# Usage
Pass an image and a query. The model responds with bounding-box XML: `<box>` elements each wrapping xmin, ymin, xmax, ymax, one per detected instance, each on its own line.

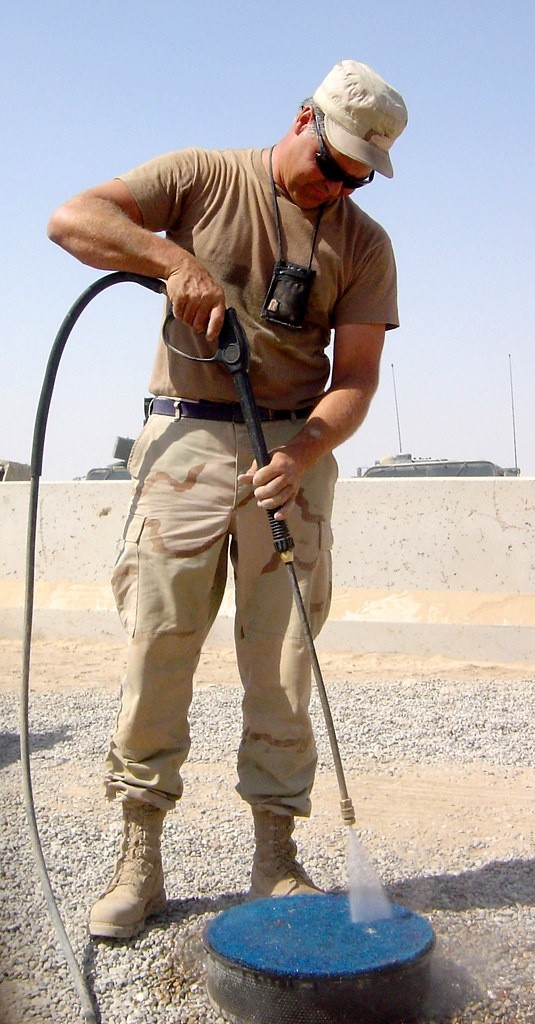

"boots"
<box><xmin>87</xmin><ymin>807</ymin><xmax>168</xmax><ymax>937</ymax></box>
<box><xmin>250</xmin><ymin>806</ymin><xmax>327</xmax><ymax>895</ymax></box>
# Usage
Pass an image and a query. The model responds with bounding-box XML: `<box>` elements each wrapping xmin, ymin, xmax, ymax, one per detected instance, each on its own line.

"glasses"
<box><xmin>302</xmin><ymin>104</ymin><xmax>375</xmax><ymax>189</ymax></box>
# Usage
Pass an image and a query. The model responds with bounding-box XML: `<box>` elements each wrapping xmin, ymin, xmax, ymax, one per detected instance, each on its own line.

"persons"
<box><xmin>47</xmin><ymin>60</ymin><xmax>409</xmax><ymax>938</ymax></box>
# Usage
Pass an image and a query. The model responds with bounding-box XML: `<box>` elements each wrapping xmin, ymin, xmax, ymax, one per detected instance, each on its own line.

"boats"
<box><xmin>359</xmin><ymin>353</ymin><xmax>524</xmax><ymax>476</ymax></box>
<box><xmin>70</xmin><ymin>459</ymin><xmax>132</xmax><ymax>480</ymax></box>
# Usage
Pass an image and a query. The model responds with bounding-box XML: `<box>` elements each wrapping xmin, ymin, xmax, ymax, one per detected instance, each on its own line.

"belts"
<box><xmin>151</xmin><ymin>399</ymin><xmax>314</xmax><ymax>423</ymax></box>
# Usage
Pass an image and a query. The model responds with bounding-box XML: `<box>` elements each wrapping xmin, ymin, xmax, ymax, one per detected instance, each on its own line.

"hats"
<box><xmin>313</xmin><ymin>60</ymin><xmax>408</xmax><ymax>178</ymax></box>
